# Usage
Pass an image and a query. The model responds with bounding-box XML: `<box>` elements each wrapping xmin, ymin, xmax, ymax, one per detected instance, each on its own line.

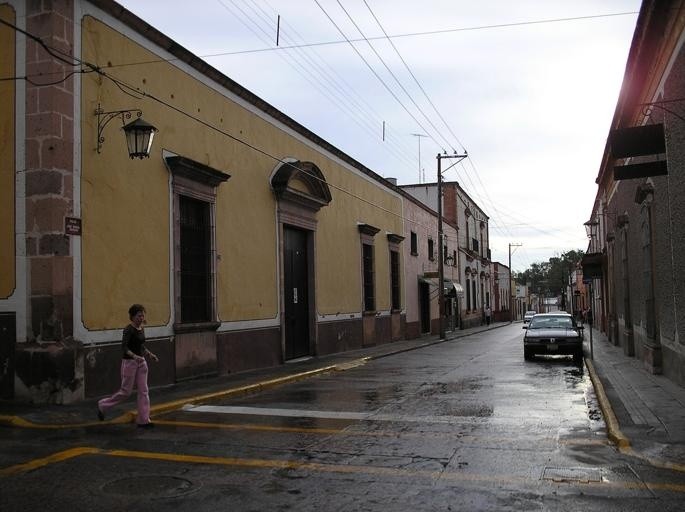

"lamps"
<box><xmin>92</xmin><ymin>103</ymin><xmax>157</xmax><ymax>160</ymax></box>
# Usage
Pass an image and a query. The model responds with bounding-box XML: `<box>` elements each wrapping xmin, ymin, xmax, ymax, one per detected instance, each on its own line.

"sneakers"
<box><xmin>136</xmin><ymin>423</ymin><xmax>154</xmax><ymax>428</ymax></box>
<box><xmin>95</xmin><ymin>400</ymin><xmax>104</xmax><ymax>421</ymax></box>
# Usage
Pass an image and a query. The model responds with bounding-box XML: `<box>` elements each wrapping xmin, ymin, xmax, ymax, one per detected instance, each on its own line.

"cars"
<box><xmin>522</xmin><ymin>310</ymin><xmax>584</xmax><ymax>362</ymax></box>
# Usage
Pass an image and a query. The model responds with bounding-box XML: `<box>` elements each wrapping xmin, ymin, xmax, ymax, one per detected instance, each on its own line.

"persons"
<box><xmin>484</xmin><ymin>306</ymin><xmax>492</xmax><ymax>326</ymax></box>
<box><xmin>94</xmin><ymin>304</ymin><xmax>160</xmax><ymax>430</ymax></box>
